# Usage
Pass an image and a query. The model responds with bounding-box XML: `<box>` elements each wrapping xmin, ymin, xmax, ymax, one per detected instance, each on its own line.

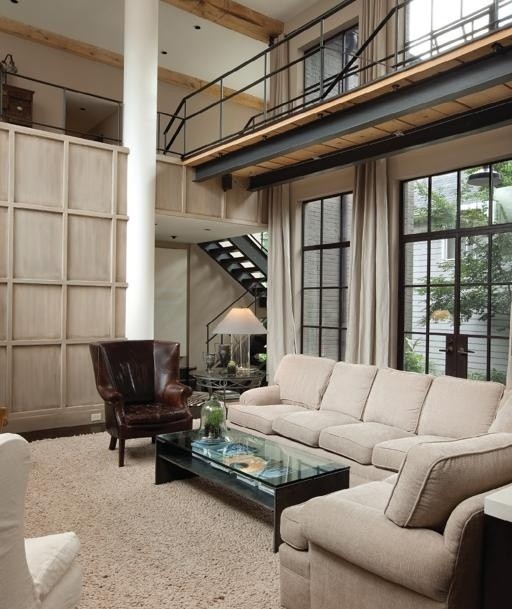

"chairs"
<box><xmin>0</xmin><ymin>431</ymin><xmax>85</xmax><ymax>609</ymax></box>
<box><xmin>87</xmin><ymin>339</ymin><xmax>193</xmax><ymax>468</ymax></box>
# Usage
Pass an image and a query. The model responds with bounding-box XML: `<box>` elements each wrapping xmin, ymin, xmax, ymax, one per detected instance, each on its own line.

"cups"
<box><xmin>218</xmin><ymin>345</ymin><xmax>230</xmax><ymax>366</ymax></box>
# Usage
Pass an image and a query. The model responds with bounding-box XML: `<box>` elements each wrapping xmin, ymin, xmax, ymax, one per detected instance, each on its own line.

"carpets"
<box><xmin>22</xmin><ymin>429</ymin><xmax>283</xmax><ymax>609</ymax></box>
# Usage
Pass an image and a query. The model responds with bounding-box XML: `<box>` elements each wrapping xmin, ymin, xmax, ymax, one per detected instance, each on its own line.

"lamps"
<box><xmin>210</xmin><ymin>308</ymin><xmax>268</xmax><ymax>371</ymax></box>
<box><xmin>468</xmin><ymin>164</ymin><xmax>502</xmax><ymax>187</ymax></box>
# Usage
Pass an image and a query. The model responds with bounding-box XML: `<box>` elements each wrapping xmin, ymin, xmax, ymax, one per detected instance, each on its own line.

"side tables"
<box><xmin>189</xmin><ymin>366</ymin><xmax>266</xmax><ymax>402</ymax></box>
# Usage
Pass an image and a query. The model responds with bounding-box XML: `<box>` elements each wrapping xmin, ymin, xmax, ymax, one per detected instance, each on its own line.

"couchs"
<box><xmin>225</xmin><ymin>353</ymin><xmax>512</xmax><ymax>609</ymax></box>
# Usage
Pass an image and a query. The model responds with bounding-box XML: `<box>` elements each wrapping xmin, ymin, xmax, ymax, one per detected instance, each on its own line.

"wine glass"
<box><xmin>206</xmin><ymin>352</ymin><xmax>216</xmax><ymax>374</ymax></box>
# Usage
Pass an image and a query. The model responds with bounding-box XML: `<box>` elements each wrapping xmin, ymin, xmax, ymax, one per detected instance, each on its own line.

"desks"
<box><xmin>484</xmin><ymin>484</ymin><xmax>512</xmax><ymax>608</ymax></box>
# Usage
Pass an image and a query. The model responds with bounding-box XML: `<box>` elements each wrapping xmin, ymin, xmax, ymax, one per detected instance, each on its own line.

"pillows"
<box><xmin>383</xmin><ymin>431</ymin><xmax>512</xmax><ymax>527</ymax></box>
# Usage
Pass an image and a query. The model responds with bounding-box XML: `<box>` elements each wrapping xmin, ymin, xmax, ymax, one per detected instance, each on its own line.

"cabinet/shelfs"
<box><xmin>0</xmin><ymin>83</ymin><xmax>36</xmax><ymax>128</ymax></box>
